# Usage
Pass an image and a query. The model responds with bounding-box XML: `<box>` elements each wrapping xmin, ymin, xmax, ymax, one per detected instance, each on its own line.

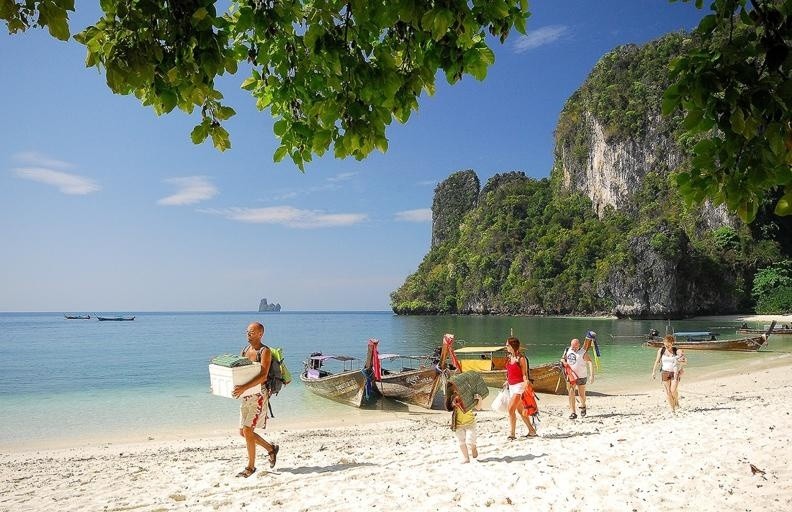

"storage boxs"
<box><xmin>208</xmin><ymin>361</ymin><xmax>263</xmax><ymax>399</ymax></box>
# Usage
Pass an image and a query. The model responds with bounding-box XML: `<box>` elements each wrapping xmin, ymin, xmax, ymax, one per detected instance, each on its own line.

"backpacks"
<box><xmin>256</xmin><ymin>345</ymin><xmax>292</xmax><ymax>396</ymax></box>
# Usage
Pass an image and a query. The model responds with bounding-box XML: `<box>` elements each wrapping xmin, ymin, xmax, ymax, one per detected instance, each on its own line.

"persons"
<box><xmin>671</xmin><ymin>348</ymin><xmax>687</xmax><ymax>384</ymax></box>
<box><xmin>561</xmin><ymin>338</ymin><xmax>594</xmax><ymax>420</ymax></box>
<box><xmin>448</xmin><ymin>392</ymin><xmax>483</xmax><ymax>464</ymax></box>
<box><xmin>502</xmin><ymin>337</ymin><xmax>540</xmax><ymax>441</ymax></box>
<box><xmin>232</xmin><ymin>322</ymin><xmax>280</xmax><ymax>479</ymax></box>
<box><xmin>650</xmin><ymin>334</ymin><xmax>688</xmax><ymax>416</ymax></box>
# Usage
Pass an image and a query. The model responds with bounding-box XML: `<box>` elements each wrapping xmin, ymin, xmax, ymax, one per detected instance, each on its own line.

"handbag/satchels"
<box><xmin>521</xmin><ymin>382</ymin><xmax>539</xmax><ymax>417</ymax></box>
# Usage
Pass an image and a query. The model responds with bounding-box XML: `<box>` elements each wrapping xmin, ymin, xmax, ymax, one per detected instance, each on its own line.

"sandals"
<box><xmin>507</xmin><ymin>434</ymin><xmax>537</xmax><ymax>440</ymax></box>
<box><xmin>581</xmin><ymin>405</ymin><xmax>586</xmax><ymax>416</ymax></box>
<box><xmin>235</xmin><ymin>466</ymin><xmax>256</xmax><ymax>478</ymax></box>
<box><xmin>569</xmin><ymin>413</ymin><xmax>577</xmax><ymax>418</ymax></box>
<box><xmin>491</xmin><ymin>385</ymin><xmax>512</xmax><ymax>415</ymax></box>
<box><xmin>268</xmin><ymin>444</ymin><xmax>278</xmax><ymax>467</ymax></box>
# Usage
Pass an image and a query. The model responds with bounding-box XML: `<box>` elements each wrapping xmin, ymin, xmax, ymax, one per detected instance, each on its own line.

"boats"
<box><xmin>297</xmin><ymin>339</ymin><xmax>380</xmax><ymax>407</ymax></box>
<box><xmin>736</xmin><ymin>323</ymin><xmax>792</xmax><ymax>334</ymax></box>
<box><xmin>64</xmin><ymin>313</ymin><xmax>91</xmax><ymax>319</ymax></box>
<box><xmin>96</xmin><ymin>316</ymin><xmax>135</xmax><ymax>321</ymax></box>
<box><xmin>452</xmin><ymin>332</ymin><xmax>599</xmax><ymax>396</ymax></box>
<box><xmin>375</xmin><ymin>333</ymin><xmax>455</xmax><ymax>409</ymax></box>
<box><xmin>643</xmin><ymin>319</ymin><xmax>777</xmax><ymax>352</ymax></box>
